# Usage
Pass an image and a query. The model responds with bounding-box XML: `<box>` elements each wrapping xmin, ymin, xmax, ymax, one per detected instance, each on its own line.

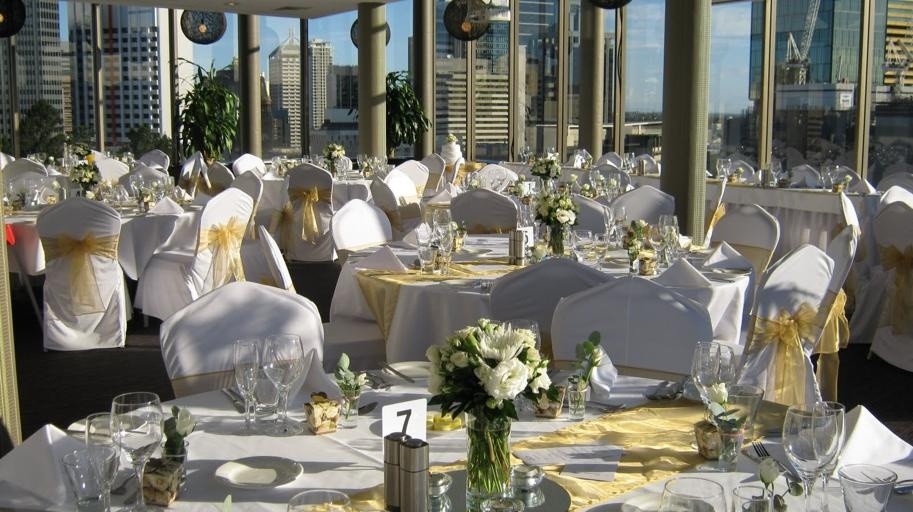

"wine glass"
<box><xmin>462</xmin><ymin>145</ymin><xmax>636</xmax><ymax>199</ymax></box>
<box><xmin>2</xmin><ymin>145</ymin><xmax>181</xmax><ymax>213</ymax></box>
<box><xmin>691</xmin><ymin>339</ymin><xmax>736</xmax><ymax>426</ymax></box>
<box><xmin>266</xmin><ymin>151</ymin><xmax>394</xmax><ymax>180</ymax></box>
<box><xmin>771</xmin><ymin>159</ymin><xmax>781</xmax><ymax>186</ymax></box>
<box><xmin>553</xmin><ymin>202</ymin><xmax>693</xmax><ymax>274</ymax></box>
<box><xmin>86</xmin><ymin>391</ymin><xmax>165</xmax><ymax>512</ymax></box>
<box><xmin>716</xmin><ymin>158</ymin><xmax>730</xmax><ymax>180</ymax></box>
<box><xmin>415</xmin><ymin>208</ymin><xmax>467</xmax><ymax>275</ymax></box>
<box><xmin>820</xmin><ymin>165</ymin><xmax>838</xmax><ymax>192</ymax></box>
<box><xmin>232</xmin><ymin>334</ymin><xmax>304</xmax><ymax>437</ymax></box>
<box><xmin>783</xmin><ymin>401</ymin><xmax>844</xmax><ymax>512</ymax></box>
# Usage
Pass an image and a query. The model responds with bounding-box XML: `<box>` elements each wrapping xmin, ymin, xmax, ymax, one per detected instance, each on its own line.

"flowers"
<box><xmin>442</xmin><ymin>134</ymin><xmax>458</xmax><ymax>144</ymax></box>
<box><xmin>320</xmin><ymin>144</ymin><xmax>345</xmax><ymax>175</ymax></box>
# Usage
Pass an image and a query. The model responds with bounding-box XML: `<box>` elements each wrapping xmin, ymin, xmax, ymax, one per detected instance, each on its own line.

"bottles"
<box><xmin>384</xmin><ymin>431</ymin><xmax>429</xmax><ymax>511</ymax></box>
<box><xmin>639</xmin><ymin>159</ymin><xmax>647</xmax><ymax>177</ymax></box>
<box><xmin>514</xmin><ymin>193</ymin><xmax>536</xmax><ymax>261</ymax></box>
<box><xmin>509</xmin><ymin>228</ymin><xmax>524</xmax><ymax>265</ymax></box>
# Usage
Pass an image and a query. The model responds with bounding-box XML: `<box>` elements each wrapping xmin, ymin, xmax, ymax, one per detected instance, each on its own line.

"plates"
<box><xmin>382</xmin><ymin>359</ymin><xmax>433</xmax><ymax>382</ymax></box>
<box><xmin>67</xmin><ymin>414</ymin><xmax>114</xmax><ymax>443</ymax></box>
<box><xmin>215</xmin><ymin>456</ymin><xmax>304</xmax><ymax>491</ymax></box>
<box><xmin>732</xmin><ymin>177</ymin><xmax>746</xmax><ymax>183</ymax></box>
<box><xmin>711</xmin><ymin>266</ymin><xmax>753</xmax><ymax>277</ymax></box>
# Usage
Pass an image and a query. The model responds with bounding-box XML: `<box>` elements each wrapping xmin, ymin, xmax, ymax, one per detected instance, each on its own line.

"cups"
<box><xmin>715</xmin><ymin>384</ymin><xmax>763</xmax><ymax>471</ymax></box>
<box><xmin>838</xmin><ymin>465</ymin><xmax>897</xmax><ymax>512</ymax></box>
<box><xmin>486</xmin><ymin>318</ymin><xmax>542</xmax><ymax>352</ymax></box>
<box><xmin>660</xmin><ymin>478</ymin><xmax>770</xmax><ymax>512</ymax></box>
<box><xmin>61</xmin><ymin>447</ymin><xmax>115</xmax><ymax>510</ymax></box>
<box><xmin>288</xmin><ymin>490</ymin><xmax>354</xmax><ymax>512</ymax></box>
<box><xmin>568</xmin><ymin>386</ymin><xmax>590</xmax><ymax>422</ymax></box>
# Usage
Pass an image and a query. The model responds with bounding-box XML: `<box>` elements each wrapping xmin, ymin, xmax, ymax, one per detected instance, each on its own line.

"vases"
<box><xmin>447</xmin><ymin>142</ymin><xmax>455</xmax><ymax>145</ymax></box>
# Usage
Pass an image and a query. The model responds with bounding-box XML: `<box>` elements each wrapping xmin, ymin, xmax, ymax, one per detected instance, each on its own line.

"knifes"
<box><xmin>221</xmin><ymin>384</ymin><xmax>255</xmax><ymax>413</ymax></box>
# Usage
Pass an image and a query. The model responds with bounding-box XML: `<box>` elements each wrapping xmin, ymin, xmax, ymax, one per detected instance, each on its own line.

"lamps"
<box><xmin>350</xmin><ymin>16</ymin><xmax>392</xmax><ymax>50</ymax></box>
<box><xmin>0</xmin><ymin>1</ymin><xmax>27</xmax><ymax>40</ymax></box>
<box><xmin>179</xmin><ymin>8</ymin><xmax>227</xmax><ymax>47</ymax></box>
<box><xmin>441</xmin><ymin>0</ymin><xmax>491</xmax><ymax>43</ymax></box>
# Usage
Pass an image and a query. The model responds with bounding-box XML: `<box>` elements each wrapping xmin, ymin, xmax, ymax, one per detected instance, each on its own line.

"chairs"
<box><xmin>0</xmin><ymin>148</ymin><xmax>913</xmax><ymax>511</ymax></box>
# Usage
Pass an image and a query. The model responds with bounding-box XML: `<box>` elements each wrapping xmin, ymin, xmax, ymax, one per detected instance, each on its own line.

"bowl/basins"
<box><xmin>429</xmin><ymin>471</ymin><xmax>454</xmax><ymax>497</ymax></box>
<box><xmin>429</xmin><ymin>494</ymin><xmax>452</xmax><ymax>512</ymax></box>
<box><xmin>478</xmin><ymin>497</ymin><xmax>525</xmax><ymax>511</ymax></box>
<box><xmin>511</xmin><ymin>465</ymin><xmax>545</xmax><ymax>490</ymax></box>
<box><xmin>511</xmin><ymin>490</ymin><xmax>545</xmax><ymax>507</ymax></box>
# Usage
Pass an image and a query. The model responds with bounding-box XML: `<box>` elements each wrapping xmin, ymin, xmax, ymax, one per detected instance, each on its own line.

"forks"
<box><xmin>754</xmin><ymin>441</ymin><xmax>802</xmax><ymax>482</ymax></box>
